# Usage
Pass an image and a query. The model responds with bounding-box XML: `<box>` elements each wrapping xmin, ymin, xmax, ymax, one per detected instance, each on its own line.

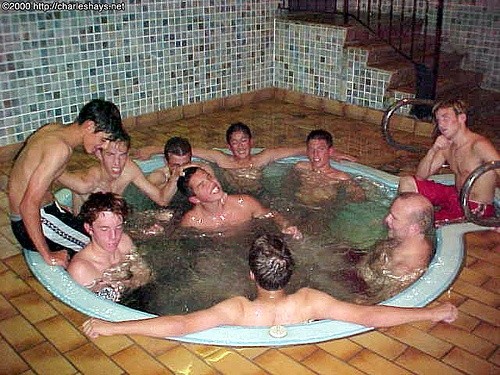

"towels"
<box><xmin>341</xmin><ymin>272</ymin><xmax>393</xmax><ymax>307</ymax></box>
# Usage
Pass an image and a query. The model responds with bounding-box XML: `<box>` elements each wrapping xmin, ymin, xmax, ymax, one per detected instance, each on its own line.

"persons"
<box><xmin>135</xmin><ymin>121</ymin><xmax>358</xmax><ymax>205</ymax></box>
<box><xmin>81</xmin><ymin>234</ymin><xmax>457</xmax><ymax>338</ymax></box>
<box><xmin>280</xmin><ymin>129</ymin><xmax>367</xmax><ymax>206</ymax></box>
<box><xmin>176</xmin><ymin>166</ymin><xmax>303</xmax><ymax>240</ymax></box>
<box><xmin>148</xmin><ymin>137</ymin><xmax>228</xmax><ymax>208</ymax></box>
<box><xmin>336</xmin><ymin>191</ymin><xmax>436</xmax><ymax>303</ymax></box>
<box><xmin>72</xmin><ymin>126</ymin><xmax>200</xmax><ymax>216</ymax></box>
<box><xmin>8</xmin><ymin>99</ymin><xmax>122</xmax><ymax>265</ymax></box>
<box><xmin>396</xmin><ymin>97</ymin><xmax>499</xmax><ymax>226</ymax></box>
<box><xmin>68</xmin><ymin>192</ymin><xmax>150</xmax><ymax>298</ymax></box>
